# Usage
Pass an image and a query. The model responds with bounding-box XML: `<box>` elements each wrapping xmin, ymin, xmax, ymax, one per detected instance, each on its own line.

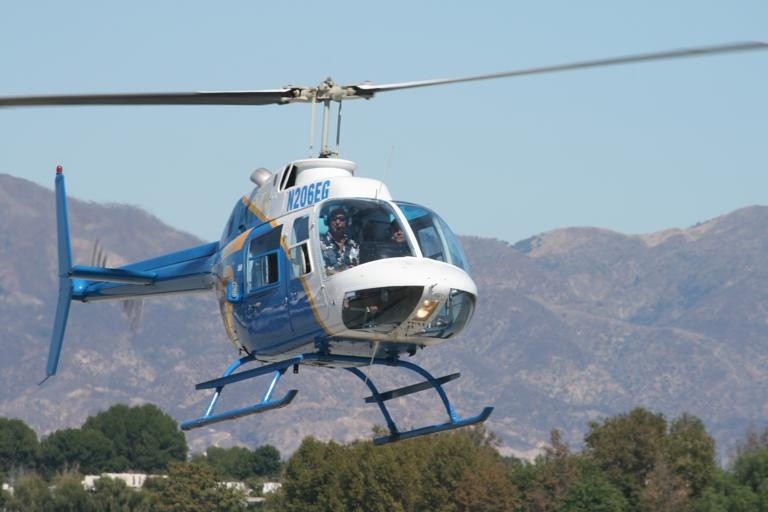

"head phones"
<box><xmin>324</xmin><ymin>203</ymin><xmax>354</xmax><ymax>227</ymax></box>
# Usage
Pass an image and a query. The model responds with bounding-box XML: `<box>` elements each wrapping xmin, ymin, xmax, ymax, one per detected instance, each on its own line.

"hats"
<box><xmin>328</xmin><ymin>203</ymin><xmax>349</xmax><ymax>223</ymax></box>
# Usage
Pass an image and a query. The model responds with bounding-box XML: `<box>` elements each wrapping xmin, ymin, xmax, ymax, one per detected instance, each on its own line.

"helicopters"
<box><xmin>0</xmin><ymin>41</ymin><xmax>768</xmax><ymax>446</ymax></box>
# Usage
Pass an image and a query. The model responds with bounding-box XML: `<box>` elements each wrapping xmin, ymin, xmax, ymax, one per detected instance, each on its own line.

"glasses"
<box><xmin>332</xmin><ymin>216</ymin><xmax>346</xmax><ymax>221</ymax></box>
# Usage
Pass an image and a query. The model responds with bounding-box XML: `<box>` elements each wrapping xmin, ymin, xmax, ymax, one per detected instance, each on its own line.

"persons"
<box><xmin>379</xmin><ymin>218</ymin><xmax>413</xmax><ymax>302</ymax></box>
<box><xmin>319</xmin><ymin>208</ymin><xmax>361</xmax><ymax>309</ymax></box>
